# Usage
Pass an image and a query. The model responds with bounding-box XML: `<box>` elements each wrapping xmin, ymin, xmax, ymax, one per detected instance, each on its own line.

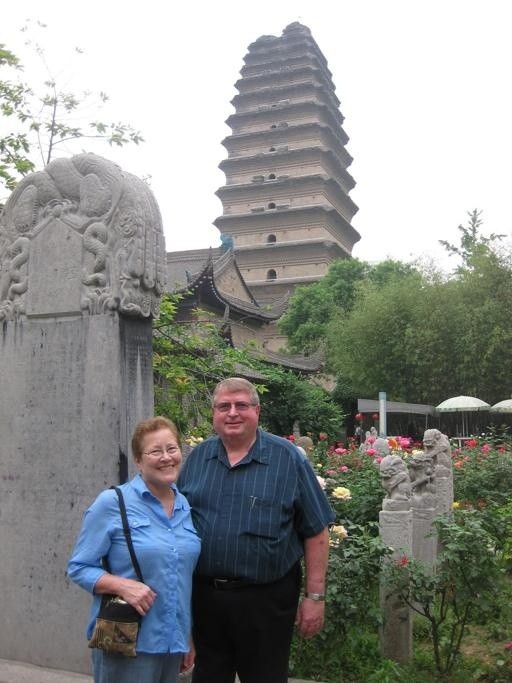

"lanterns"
<box><xmin>355</xmin><ymin>413</ymin><xmax>367</xmax><ymax>424</ymax></box>
<box><xmin>372</xmin><ymin>412</ymin><xmax>379</xmax><ymax>422</ymax></box>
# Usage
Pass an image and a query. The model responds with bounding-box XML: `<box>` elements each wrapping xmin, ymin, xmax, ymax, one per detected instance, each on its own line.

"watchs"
<box><xmin>304</xmin><ymin>591</ymin><xmax>325</xmax><ymax>601</ymax></box>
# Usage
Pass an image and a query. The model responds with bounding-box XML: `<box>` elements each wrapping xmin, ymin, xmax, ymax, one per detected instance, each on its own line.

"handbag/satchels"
<box><xmin>88</xmin><ymin>594</ymin><xmax>142</xmax><ymax>658</ymax></box>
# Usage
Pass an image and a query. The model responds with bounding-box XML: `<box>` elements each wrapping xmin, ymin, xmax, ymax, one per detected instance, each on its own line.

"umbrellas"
<box><xmin>435</xmin><ymin>395</ymin><xmax>490</xmax><ymax>437</ymax></box>
<box><xmin>488</xmin><ymin>398</ymin><xmax>512</xmax><ymax>414</ymax></box>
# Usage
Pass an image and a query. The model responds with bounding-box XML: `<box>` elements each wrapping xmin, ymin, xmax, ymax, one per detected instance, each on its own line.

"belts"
<box><xmin>192</xmin><ymin>573</ymin><xmax>267</xmax><ymax>590</ymax></box>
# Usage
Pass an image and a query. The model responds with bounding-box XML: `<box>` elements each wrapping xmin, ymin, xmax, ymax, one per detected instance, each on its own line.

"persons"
<box><xmin>176</xmin><ymin>377</ymin><xmax>336</xmax><ymax>683</ymax></box>
<box><xmin>66</xmin><ymin>415</ymin><xmax>203</xmax><ymax>683</ymax></box>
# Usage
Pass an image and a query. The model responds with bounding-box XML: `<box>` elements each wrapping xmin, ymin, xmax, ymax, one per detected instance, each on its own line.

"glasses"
<box><xmin>213</xmin><ymin>402</ymin><xmax>257</xmax><ymax>412</ymax></box>
<box><xmin>138</xmin><ymin>444</ymin><xmax>180</xmax><ymax>459</ymax></box>
